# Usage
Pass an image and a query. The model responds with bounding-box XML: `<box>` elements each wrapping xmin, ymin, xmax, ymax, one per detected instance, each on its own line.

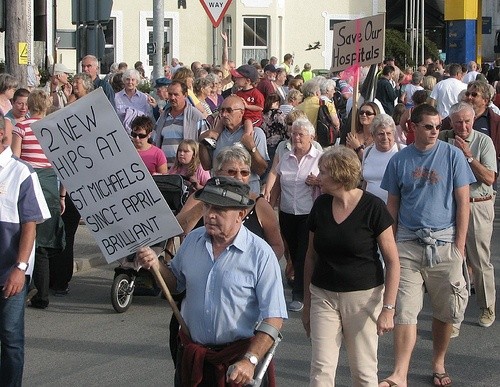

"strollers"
<box><xmin>111</xmin><ymin>173</ymin><xmax>204</xmax><ymax>313</ymax></box>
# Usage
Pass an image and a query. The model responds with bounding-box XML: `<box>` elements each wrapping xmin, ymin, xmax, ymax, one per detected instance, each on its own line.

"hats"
<box><xmin>153</xmin><ymin>78</ymin><xmax>173</xmax><ymax>87</ymax></box>
<box><xmin>229</xmin><ymin>65</ymin><xmax>258</xmax><ymax>82</ymax></box>
<box><xmin>49</xmin><ymin>64</ymin><xmax>73</xmax><ymax>75</ymax></box>
<box><xmin>193</xmin><ymin>177</ymin><xmax>255</xmax><ymax>209</ymax></box>
<box><xmin>264</xmin><ymin>65</ymin><xmax>279</xmax><ymax>72</ymax></box>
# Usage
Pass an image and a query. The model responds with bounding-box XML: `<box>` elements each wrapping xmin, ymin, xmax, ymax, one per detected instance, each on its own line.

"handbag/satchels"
<box><xmin>316</xmin><ymin>98</ymin><xmax>338</xmax><ymax>147</ymax></box>
<box><xmin>333</xmin><ymin>79</ymin><xmax>348</xmax><ymax>118</ymax></box>
<box><xmin>112</xmin><ymin>72</ymin><xmax>124</xmax><ymax>92</ymax></box>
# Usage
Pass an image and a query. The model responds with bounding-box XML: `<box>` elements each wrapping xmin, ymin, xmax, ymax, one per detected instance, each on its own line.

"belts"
<box><xmin>470</xmin><ymin>195</ymin><xmax>492</xmax><ymax>202</ymax></box>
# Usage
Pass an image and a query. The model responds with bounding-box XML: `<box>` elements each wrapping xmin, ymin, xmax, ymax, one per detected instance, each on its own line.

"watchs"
<box><xmin>467</xmin><ymin>155</ymin><xmax>475</xmax><ymax>163</ymax></box>
<box><xmin>14</xmin><ymin>262</ymin><xmax>29</xmax><ymax>272</ymax></box>
<box><xmin>244</xmin><ymin>352</ymin><xmax>259</xmax><ymax>366</ymax></box>
<box><xmin>248</xmin><ymin>146</ymin><xmax>257</xmax><ymax>155</ymax></box>
<box><xmin>383</xmin><ymin>304</ymin><xmax>395</xmax><ymax>310</ymax></box>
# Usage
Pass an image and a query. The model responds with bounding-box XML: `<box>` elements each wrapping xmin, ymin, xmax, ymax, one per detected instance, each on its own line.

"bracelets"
<box><xmin>354</xmin><ymin>145</ymin><xmax>364</xmax><ymax>153</ymax></box>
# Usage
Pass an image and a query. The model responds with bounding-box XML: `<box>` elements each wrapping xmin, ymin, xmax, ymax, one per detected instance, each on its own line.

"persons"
<box><xmin>137</xmin><ymin>174</ymin><xmax>289</xmax><ymax>387</ymax></box>
<box><xmin>302</xmin><ymin>145</ymin><xmax>400</xmax><ymax>387</ymax></box>
<box><xmin>0</xmin><ymin>32</ymin><xmax>500</xmax><ymax>310</ymax></box>
<box><xmin>378</xmin><ymin>104</ymin><xmax>477</xmax><ymax>387</ymax></box>
<box><xmin>437</xmin><ymin>102</ymin><xmax>498</xmax><ymax>339</ymax></box>
<box><xmin>0</xmin><ymin>111</ymin><xmax>52</xmax><ymax>387</ymax></box>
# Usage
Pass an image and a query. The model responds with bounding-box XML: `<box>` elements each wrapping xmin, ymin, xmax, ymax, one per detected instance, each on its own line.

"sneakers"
<box><xmin>287</xmin><ymin>300</ymin><xmax>304</xmax><ymax>312</ymax></box>
<box><xmin>478</xmin><ymin>303</ymin><xmax>496</xmax><ymax>327</ymax></box>
<box><xmin>449</xmin><ymin>327</ymin><xmax>459</xmax><ymax>338</ymax></box>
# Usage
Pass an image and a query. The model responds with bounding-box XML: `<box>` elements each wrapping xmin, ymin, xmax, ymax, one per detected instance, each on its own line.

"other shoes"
<box><xmin>233</xmin><ymin>142</ymin><xmax>247</xmax><ymax>149</ymax></box>
<box><xmin>285</xmin><ymin>263</ymin><xmax>295</xmax><ymax>286</ymax></box>
<box><xmin>55</xmin><ymin>288</ymin><xmax>71</xmax><ymax>297</ymax></box>
<box><xmin>26</xmin><ymin>283</ymin><xmax>38</xmax><ymax>304</ymax></box>
<box><xmin>204</xmin><ymin>137</ymin><xmax>217</xmax><ymax>148</ymax></box>
<box><xmin>28</xmin><ymin>298</ymin><xmax>48</xmax><ymax>305</ymax></box>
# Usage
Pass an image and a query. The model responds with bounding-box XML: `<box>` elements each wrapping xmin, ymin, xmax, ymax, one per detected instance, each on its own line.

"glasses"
<box><xmin>291</xmin><ymin>132</ymin><xmax>308</xmax><ymax>137</ymax></box>
<box><xmin>131</xmin><ymin>131</ymin><xmax>149</xmax><ymax>138</ymax></box>
<box><xmin>416</xmin><ymin>124</ymin><xmax>441</xmax><ymax>130</ymax></box>
<box><xmin>465</xmin><ymin>92</ymin><xmax>483</xmax><ymax>97</ymax></box>
<box><xmin>359</xmin><ymin>111</ymin><xmax>375</xmax><ymax>115</ymax></box>
<box><xmin>219</xmin><ymin>108</ymin><xmax>241</xmax><ymax>113</ymax></box>
<box><xmin>222</xmin><ymin>168</ymin><xmax>250</xmax><ymax>177</ymax></box>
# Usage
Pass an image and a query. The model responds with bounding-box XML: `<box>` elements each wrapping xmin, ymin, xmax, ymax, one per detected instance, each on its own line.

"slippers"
<box><xmin>430</xmin><ymin>371</ymin><xmax>453</xmax><ymax>387</ymax></box>
<box><xmin>376</xmin><ymin>378</ymin><xmax>408</xmax><ymax>387</ymax></box>
